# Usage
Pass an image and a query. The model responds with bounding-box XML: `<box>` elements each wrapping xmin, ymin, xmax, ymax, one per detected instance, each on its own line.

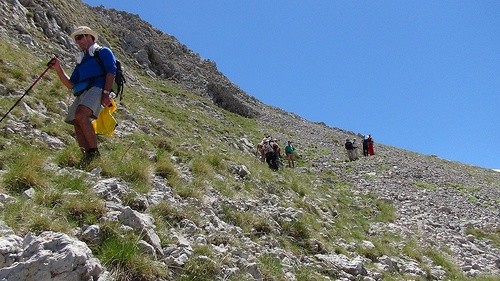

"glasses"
<box><xmin>74</xmin><ymin>35</ymin><xmax>85</xmax><ymax>41</ymax></box>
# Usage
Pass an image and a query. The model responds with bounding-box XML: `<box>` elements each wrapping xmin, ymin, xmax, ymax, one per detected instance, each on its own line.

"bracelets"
<box><xmin>103</xmin><ymin>88</ymin><xmax>111</xmax><ymax>91</ymax></box>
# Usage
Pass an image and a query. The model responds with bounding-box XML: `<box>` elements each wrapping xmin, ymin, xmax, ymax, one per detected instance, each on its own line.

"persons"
<box><xmin>48</xmin><ymin>26</ymin><xmax>126</xmax><ymax>170</ymax></box>
<box><xmin>285</xmin><ymin>141</ymin><xmax>296</xmax><ymax>168</ymax></box>
<box><xmin>255</xmin><ymin>133</ymin><xmax>285</xmax><ymax>172</ymax></box>
<box><xmin>345</xmin><ymin>134</ymin><xmax>374</xmax><ymax>162</ymax></box>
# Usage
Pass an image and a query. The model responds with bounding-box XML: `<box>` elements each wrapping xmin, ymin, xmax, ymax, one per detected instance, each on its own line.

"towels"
<box><xmin>75</xmin><ymin>43</ymin><xmax>102</xmax><ymax>64</ymax></box>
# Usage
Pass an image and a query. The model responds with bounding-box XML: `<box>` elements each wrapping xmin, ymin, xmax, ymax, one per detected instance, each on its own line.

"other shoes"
<box><xmin>76</xmin><ymin>149</ymin><xmax>102</xmax><ymax>170</ymax></box>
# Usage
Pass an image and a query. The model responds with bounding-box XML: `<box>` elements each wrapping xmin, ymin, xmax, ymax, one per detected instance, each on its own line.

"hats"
<box><xmin>70</xmin><ymin>26</ymin><xmax>105</xmax><ymax>41</ymax></box>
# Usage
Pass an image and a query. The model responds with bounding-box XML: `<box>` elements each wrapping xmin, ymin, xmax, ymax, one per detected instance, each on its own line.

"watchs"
<box><xmin>102</xmin><ymin>90</ymin><xmax>110</xmax><ymax>94</ymax></box>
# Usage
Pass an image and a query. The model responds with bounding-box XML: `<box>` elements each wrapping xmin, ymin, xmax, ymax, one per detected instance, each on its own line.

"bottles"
<box><xmin>99</xmin><ymin>92</ymin><xmax>116</xmax><ymax>110</ymax></box>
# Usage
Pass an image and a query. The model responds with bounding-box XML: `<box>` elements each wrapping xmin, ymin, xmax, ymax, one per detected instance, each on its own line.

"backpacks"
<box><xmin>93</xmin><ymin>46</ymin><xmax>126</xmax><ymax>102</ymax></box>
